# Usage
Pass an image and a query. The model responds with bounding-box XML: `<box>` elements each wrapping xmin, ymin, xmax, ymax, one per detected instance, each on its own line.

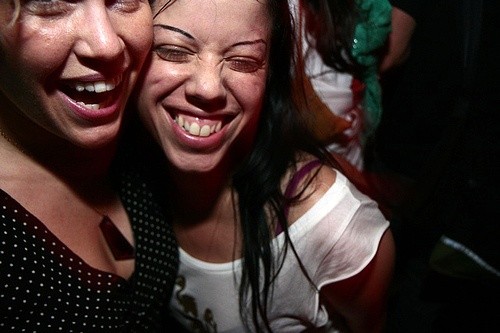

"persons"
<box><xmin>147</xmin><ymin>0</ymin><xmax>396</xmax><ymax>332</ymax></box>
<box><xmin>0</xmin><ymin>1</ymin><xmax>185</xmax><ymax>333</ymax></box>
